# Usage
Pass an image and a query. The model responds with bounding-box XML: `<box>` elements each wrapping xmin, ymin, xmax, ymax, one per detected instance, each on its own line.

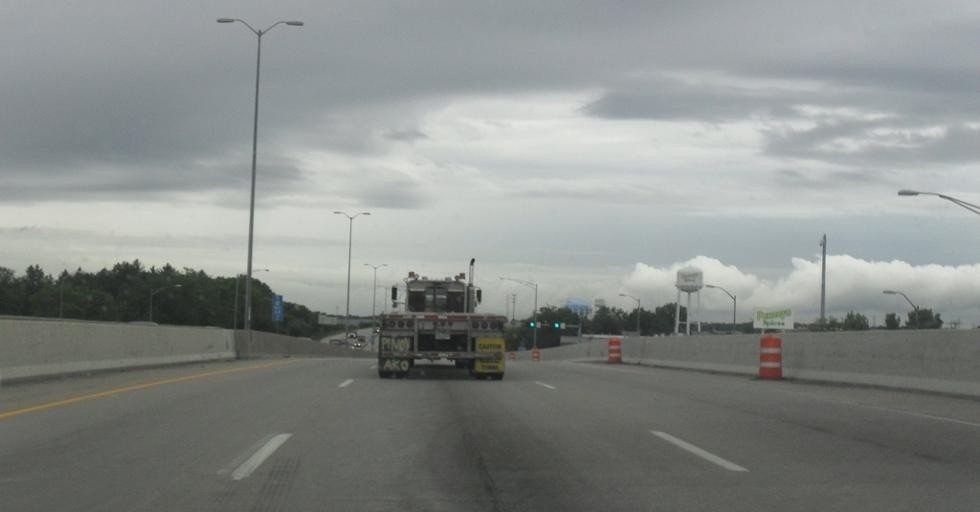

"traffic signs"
<box><xmin>883</xmin><ymin>290</ymin><xmax>921</xmax><ymax>317</ymax></box>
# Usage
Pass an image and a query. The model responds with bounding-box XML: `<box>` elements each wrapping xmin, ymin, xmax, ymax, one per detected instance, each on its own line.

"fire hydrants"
<box><xmin>233</xmin><ymin>268</ymin><xmax>271</xmax><ymax>328</ymax></box>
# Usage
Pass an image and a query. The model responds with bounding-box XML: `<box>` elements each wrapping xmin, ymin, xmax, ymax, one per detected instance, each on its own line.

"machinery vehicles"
<box><xmin>372</xmin><ymin>255</ymin><xmax>511</xmax><ymax>380</ymax></box>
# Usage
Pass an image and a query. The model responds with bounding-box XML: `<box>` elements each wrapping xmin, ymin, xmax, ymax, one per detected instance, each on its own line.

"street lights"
<box><xmin>216</xmin><ymin>16</ymin><xmax>304</xmax><ymax>330</ymax></box>
<box><xmin>499</xmin><ymin>274</ymin><xmax>538</xmax><ymax>349</ymax></box>
<box><xmin>618</xmin><ymin>292</ymin><xmax>641</xmax><ymax>337</ymax></box>
<box><xmin>331</xmin><ymin>209</ymin><xmax>371</xmax><ymax>345</ymax></box>
<box><xmin>704</xmin><ymin>284</ymin><xmax>737</xmax><ymax>336</ymax></box>
<box><xmin>149</xmin><ymin>283</ymin><xmax>182</xmax><ymax>321</ymax></box>
